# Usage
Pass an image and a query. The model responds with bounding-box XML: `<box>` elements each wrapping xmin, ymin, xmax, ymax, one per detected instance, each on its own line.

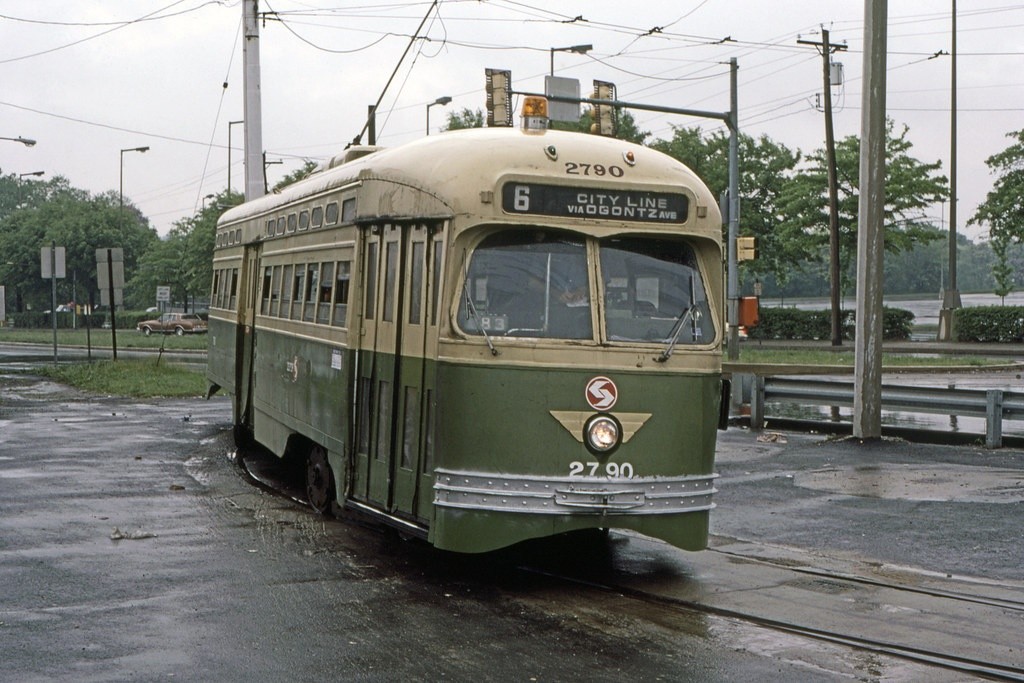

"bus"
<box><xmin>206</xmin><ymin>125</ymin><xmax>724</xmax><ymax>554</ymax></box>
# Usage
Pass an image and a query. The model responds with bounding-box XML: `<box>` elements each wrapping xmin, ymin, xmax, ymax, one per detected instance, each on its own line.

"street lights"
<box><xmin>426</xmin><ymin>97</ymin><xmax>452</xmax><ymax>133</ymax></box>
<box><xmin>120</xmin><ymin>147</ymin><xmax>149</xmax><ymax>240</ymax></box>
<box><xmin>551</xmin><ymin>44</ymin><xmax>593</xmax><ymax>79</ymax></box>
<box><xmin>19</xmin><ymin>171</ymin><xmax>44</xmax><ymax>206</ymax></box>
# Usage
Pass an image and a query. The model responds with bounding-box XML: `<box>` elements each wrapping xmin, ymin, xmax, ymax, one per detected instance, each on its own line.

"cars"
<box><xmin>138</xmin><ymin>312</ymin><xmax>208</xmax><ymax>336</ymax></box>
